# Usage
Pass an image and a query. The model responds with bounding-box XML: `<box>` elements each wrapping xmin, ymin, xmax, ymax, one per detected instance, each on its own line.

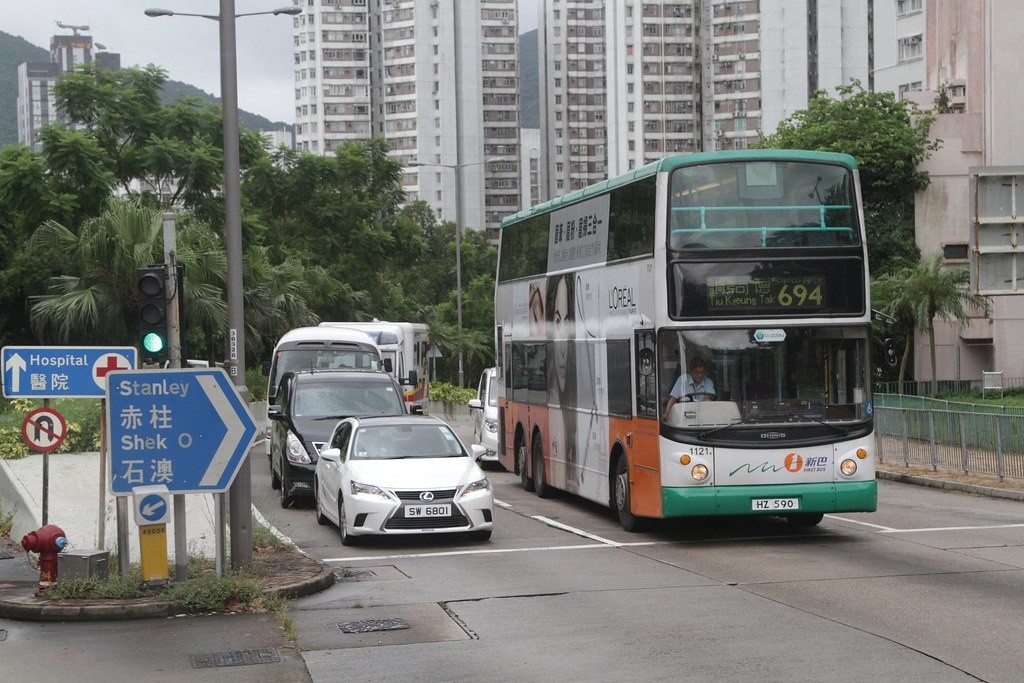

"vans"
<box><xmin>268</xmin><ymin>368</ymin><xmax>410</xmax><ymax>508</ymax></box>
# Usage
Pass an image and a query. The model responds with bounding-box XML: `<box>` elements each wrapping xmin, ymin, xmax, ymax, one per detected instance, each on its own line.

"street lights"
<box><xmin>408</xmin><ymin>157</ymin><xmax>503</xmax><ymax>390</ymax></box>
<box><xmin>142</xmin><ymin>6</ymin><xmax>303</xmax><ymax>573</ymax></box>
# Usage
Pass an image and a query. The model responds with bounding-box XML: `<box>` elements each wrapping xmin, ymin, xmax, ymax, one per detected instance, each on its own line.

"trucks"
<box><xmin>321</xmin><ymin>318</ymin><xmax>433</xmax><ymax>415</ymax></box>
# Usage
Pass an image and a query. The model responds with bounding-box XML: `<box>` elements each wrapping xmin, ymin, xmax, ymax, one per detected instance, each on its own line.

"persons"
<box><xmin>545</xmin><ymin>273</ymin><xmax>580</xmax><ymax>495</ymax></box>
<box><xmin>662</xmin><ymin>357</ymin><xmax>715</xmax><ymax>421</ymax></box>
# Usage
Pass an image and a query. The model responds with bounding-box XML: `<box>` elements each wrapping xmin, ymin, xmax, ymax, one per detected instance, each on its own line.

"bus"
<box><xmin>495</xmin><ymin>148</ymin><xmax>879</xmax><ymax>533</ymax></box>
<box><xmin>267</xmin><ymin>326</ymin><xmax>385</xmax><ymax>469</ymax></box>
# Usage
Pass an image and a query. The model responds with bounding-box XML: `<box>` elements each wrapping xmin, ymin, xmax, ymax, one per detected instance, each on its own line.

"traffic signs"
<box><xmin>106</xmin><ymin>367</ymin><xmax>259</xmax><ymax>496</ymax></box>
<box><xmin>1</xmin><ymin>345</ymin><xmax>142</xmax><ymax>399</ymax></box>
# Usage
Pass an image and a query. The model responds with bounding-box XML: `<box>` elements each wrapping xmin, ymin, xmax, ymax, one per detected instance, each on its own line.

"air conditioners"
<box><xmin>948</xmin><ymin>87</ymin><xmax>964</xmax><ymax>106</ymax></box>
<box><xmin>905</xmin><ymin>37</ymin><xmax>918</xmax><ymax>45</ymax></box>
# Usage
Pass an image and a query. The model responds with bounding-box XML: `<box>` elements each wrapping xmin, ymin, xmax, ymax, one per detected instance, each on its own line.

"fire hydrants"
<box><xmin>20</xmin><ymin>525</ymin><xmax>68</xmax><ymax>598</ymax></box>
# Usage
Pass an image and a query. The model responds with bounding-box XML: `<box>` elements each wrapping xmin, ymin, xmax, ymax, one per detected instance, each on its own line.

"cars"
<box><xmin>315</xmin><ymin>414</ymin><xmax>495</xmax><ymax>550</ymax></box>
<box><xmin>469</xmin><ymin>368</ymin><xmax>499</xmax><ymax>469</ymax></box>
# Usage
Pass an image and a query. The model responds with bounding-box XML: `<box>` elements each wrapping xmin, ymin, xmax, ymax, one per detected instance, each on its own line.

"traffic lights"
<box><xmin>132</xmin><ymin>265</ymin><xmax>174</xmax><ymax>362</ymax></box>
